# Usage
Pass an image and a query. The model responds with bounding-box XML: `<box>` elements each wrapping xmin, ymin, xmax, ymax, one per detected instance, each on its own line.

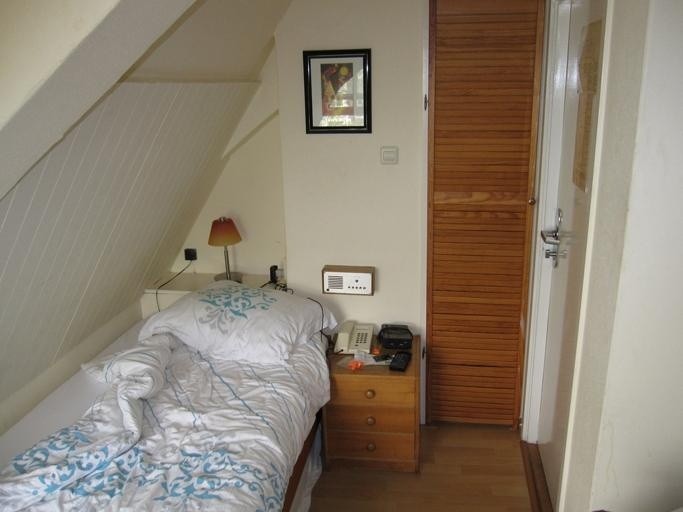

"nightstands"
<box><xmin>321</xmin><ymin>334</ymin><xmax>420</xmax><ymax>476</ymax></box>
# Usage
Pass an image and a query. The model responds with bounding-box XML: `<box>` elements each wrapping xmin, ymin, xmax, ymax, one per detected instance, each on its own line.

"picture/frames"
<box><xmin>301</xmin><ymin>47</ymin><xmax>372</xmax><ymax>135</ymax></box>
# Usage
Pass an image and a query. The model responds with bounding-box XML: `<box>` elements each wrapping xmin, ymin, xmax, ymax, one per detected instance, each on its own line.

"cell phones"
<box><xmin>388</xmin><ymin>351</ymin><xmax>413</xmax><ymax>372</ymax></box>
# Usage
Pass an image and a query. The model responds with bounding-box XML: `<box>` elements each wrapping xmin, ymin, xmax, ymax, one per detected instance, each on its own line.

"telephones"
<box><xmin>334</xmin><ymin>320</ymin><xmax>374</xmax><ymax>355</ymax></box>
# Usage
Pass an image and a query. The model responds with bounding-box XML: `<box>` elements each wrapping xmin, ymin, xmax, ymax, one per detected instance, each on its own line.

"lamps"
<box><xmin>206</xmin><ymin>217</ymin><xmax>244</xmax><ymax>283</ymax></box>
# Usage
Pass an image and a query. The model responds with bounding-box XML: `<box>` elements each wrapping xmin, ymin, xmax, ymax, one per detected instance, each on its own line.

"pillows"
<box><xmin>137</xmin><ymin>279</ymin><xmax>337</xmax><ymax>368</ymax></box>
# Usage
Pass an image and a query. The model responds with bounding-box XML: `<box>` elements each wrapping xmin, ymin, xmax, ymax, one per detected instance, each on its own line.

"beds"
<box><xmin>0</xmin><ymin>318</ymin><xmax>332</xmax><ymax>511</ymax></box>
